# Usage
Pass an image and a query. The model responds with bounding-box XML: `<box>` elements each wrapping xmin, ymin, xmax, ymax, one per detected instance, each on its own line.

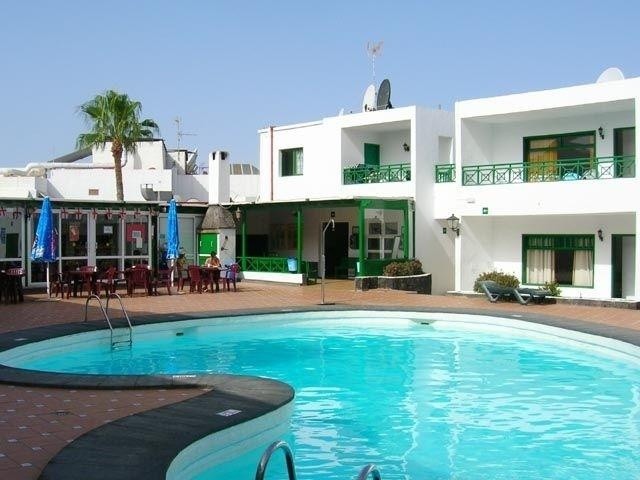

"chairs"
<box><xmin>349</xmin><ymin>162</ymin><xmax>410</xmax><ymax>182</ymax></box>
<box><xmin>300</xmin><ymin>258</ymin><xmax>318</xmax><ymax>285</ymax></box>
<box><xmin>0</xmin><ymin>259</ymin><xmax>241</xmax><ymax>303</ymax></box>
<box><xmin>479</xmin><ymin>279</ymin><xmax>562</xmax><ymax>305</ymax></box>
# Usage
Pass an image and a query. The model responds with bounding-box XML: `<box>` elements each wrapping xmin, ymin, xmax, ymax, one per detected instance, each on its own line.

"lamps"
<box><xmin>235</xmin><ymin>207</ymin><xmax>245</xmax><ymax>222</ymax></box>
<box><xmin>400</xmin><ymin>135</ymin><xmax>409</xmax><ymax>152</ymax></box>
<box><xmin>596</xmin><ymin>228</ymin><xmax>604</xmax><ymax>242</ymax></box>
<box><xmin>446</xmin><ymin>214</ymin><xmax>460</xmax><ymax>238</ymax></box>
<box><xmin>598</xmin><ymin>126</ymin><xmax>605</xmax><ymax>140</ymax></box>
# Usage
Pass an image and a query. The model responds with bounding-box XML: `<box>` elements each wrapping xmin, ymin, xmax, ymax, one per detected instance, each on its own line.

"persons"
<box><xmin>202</xmin><ymin>251</ymin><xmax>221</xmax><ymax>291</ymax></box>
<box><xmin>176</xmin><ymin>249</ymin><xmax>189</xmax><ymax>295</ymax></box>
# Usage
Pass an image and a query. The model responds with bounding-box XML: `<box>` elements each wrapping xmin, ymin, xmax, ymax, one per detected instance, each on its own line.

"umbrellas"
<box><xmin>164</xmin><ymin>198</ymin><xmax>180</xmax><ymax>292</ymax></box>
<box><xmin>29</xmin><ymin>196</ymin><xmax>57</xmax><ymax>299</ymax></box>
